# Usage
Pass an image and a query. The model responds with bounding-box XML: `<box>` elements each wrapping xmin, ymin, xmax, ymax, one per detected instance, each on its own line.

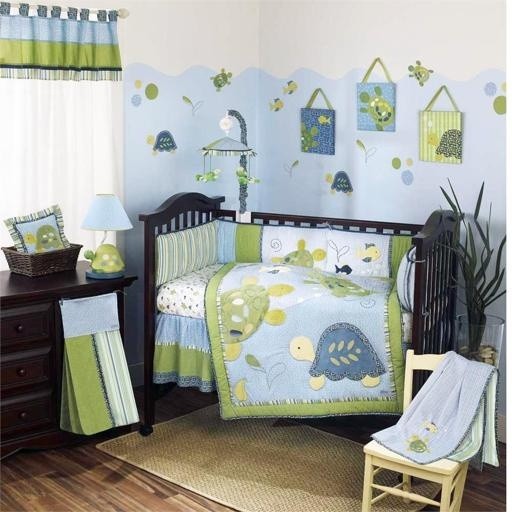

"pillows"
<box><xmin>396</xmin><ymin>246</ymin><xmax>449</xmax><ymax>311</ymax></box>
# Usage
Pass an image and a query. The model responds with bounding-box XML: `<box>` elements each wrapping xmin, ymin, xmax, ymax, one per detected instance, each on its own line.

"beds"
<box><xmin>139</xmin><ymin>192</ymin><xmax>464</xmax><ymax>484</ymax></box>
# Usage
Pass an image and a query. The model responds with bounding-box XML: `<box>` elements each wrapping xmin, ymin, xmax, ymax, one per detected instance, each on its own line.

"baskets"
<box><xmin>0</xmin><ymin>244</ymin><xmax>83</xmax><ymax>278</ymax></box>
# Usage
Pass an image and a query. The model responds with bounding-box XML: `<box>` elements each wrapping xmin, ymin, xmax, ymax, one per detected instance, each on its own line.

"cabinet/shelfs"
<box><xmin>1</xmin><ymin>261</ymin><xmax>138</xmax><ymax>462</ymax></box>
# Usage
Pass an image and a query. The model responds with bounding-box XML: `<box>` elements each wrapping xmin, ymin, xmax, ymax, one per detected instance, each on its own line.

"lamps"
<box><xmin>82</xmin><ymin>194</ymin><xmax>133</xmax><ymax>280</ymax></box>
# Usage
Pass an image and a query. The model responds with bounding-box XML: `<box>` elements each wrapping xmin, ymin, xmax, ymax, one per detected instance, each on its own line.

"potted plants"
<box><xmin>435</xmin><ymin>178</ymin><xmax>504</xmax><ymax>367</ymax></box>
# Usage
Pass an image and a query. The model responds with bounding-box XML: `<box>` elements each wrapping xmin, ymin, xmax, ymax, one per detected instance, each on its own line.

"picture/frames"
<box><xmin>6</xmin><ymin>204</ymin><xmax>71</xmax><ymax>254</ymax></box>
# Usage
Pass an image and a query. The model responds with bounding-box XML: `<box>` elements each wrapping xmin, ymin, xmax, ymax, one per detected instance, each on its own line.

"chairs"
<box><xmin>361</xmin><ymin>349</ymin><xmax>475</xmax><ymax>512</ymax></box>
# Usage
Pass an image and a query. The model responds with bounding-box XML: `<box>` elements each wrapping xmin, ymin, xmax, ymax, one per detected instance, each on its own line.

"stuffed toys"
<box><xmin>235</xmin><ymin>166</ymin><xmax>260</xmax><ymax>185</ymax></box>
<box><xmin>204</xmin><ymin>168</ymin><xmax>222</xmax><ymax>183</ymax></box>
<box><xmin>195</xmin><ymin>172</ymin><xmax>208</xmax><ymax>184</ymax></box>
<box><xmin>84</xmin><ymin>243</ymin><xmax>126</xmax><ymax>273</ymax></box>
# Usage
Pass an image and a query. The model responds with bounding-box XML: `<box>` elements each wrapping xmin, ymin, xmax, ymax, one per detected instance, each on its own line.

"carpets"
<box><xmin>95</xmin><ymin>400</ymin><xmax>442</xmax><ymax>511</ymax></box>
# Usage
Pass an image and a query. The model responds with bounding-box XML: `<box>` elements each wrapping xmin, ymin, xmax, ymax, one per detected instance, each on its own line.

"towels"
<box><xmin>370</xmin><ymin>351</ymin><xmax>499</xmax><ymax>473</ymax></box>
<box><xmin>60</xmin><ymin>291</ymin><xmax>141</xmax><ymax>435</ymax></box>
